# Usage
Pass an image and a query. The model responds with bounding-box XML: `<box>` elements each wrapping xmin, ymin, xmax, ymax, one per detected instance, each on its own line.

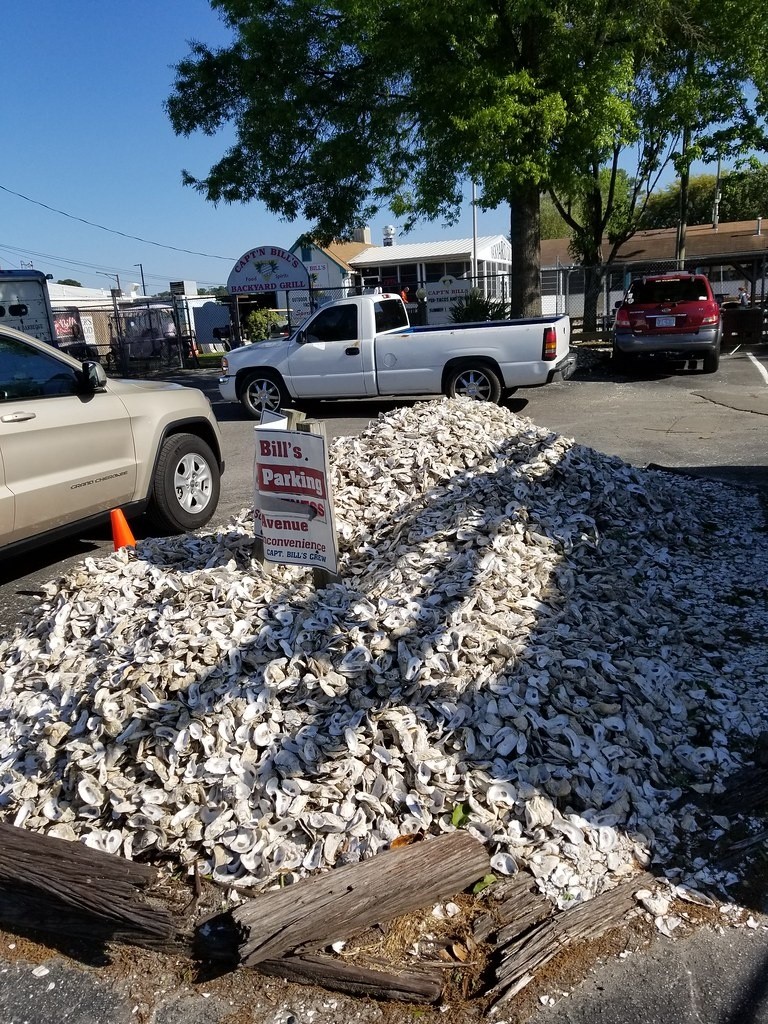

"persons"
<box><xmin>70</xmin><ymin>322</ymin><xmax>85</xmax><ymax>341</ymax></box>
<box><xmin>399</xmin><ymin>286</ymin><xmax>410</xmax><ymax>305</ymax></box>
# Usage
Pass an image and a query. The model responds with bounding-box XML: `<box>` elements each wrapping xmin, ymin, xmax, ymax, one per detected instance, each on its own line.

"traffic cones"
<box><xmin>110</xmin><ymin>510</ymin><xmax>136</xmax><ymax>553</ymax></box>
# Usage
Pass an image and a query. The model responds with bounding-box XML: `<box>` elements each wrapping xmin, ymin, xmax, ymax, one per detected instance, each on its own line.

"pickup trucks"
<box><xmin>218</xmin><ymin>292</ymin><xmax>576</xmax><ymax>421</ymax></box>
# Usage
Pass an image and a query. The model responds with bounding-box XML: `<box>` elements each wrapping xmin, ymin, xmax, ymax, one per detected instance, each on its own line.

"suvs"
<box><xmin>614</xmin><ymin>274</ymin><xmax>724</xmax><ymax>376</ymax></box>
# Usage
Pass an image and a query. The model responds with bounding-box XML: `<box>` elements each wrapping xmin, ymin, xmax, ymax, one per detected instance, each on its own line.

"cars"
<box><xmin>0</xmin><ymin>322</ymin><xmax>224</xmax><ymax>560</ymax></box>
